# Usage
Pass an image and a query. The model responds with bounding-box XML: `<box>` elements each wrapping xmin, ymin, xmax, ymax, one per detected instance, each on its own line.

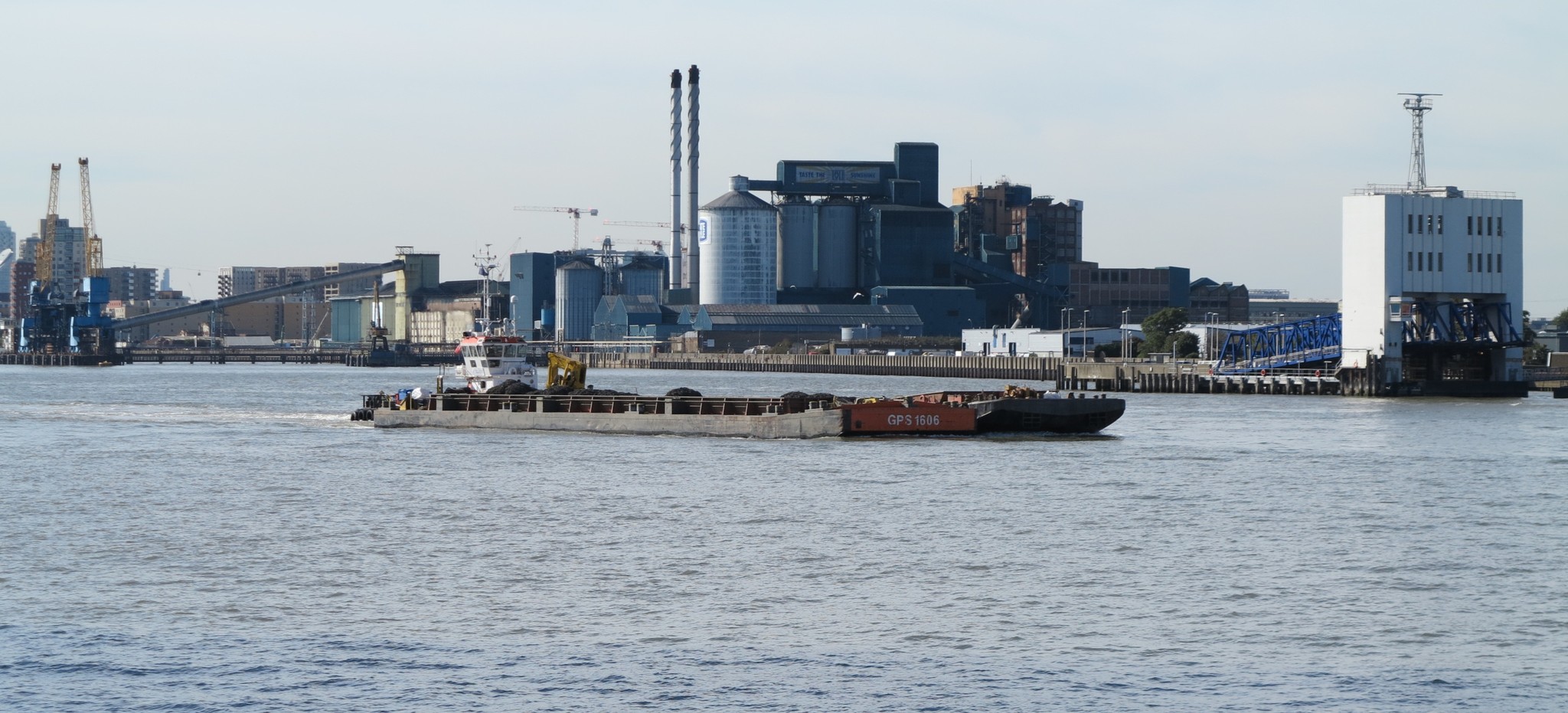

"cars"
<box><xmin>1176</xmin><ymin>360</ymin><xmax>1193</xmax><ymax>366</ymax></box>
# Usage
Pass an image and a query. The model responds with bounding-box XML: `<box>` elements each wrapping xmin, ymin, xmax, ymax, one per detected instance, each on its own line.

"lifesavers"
<box><xmin>1353</xmin><ymin>362</ymin><xmax>1358</xmax><ymax>368</ymax></box>
<box><xmin>1261</xmin><ymin>369</ymin><xmax>1266</xmax><ymax>376</ymax></box>
<box><xmin>350</xmin><ymin>409</ymin><xmax>373</xmax><ymax>422</ymax></box>
<box><xmin>1208</xmin><ymin>369</ymin><xmax>1213</xmax><ymax>375</ymax></box>
<box><xmin>1315</xmin><ymin>370</ymin><xmax>1321</xmax><ymax>377</ymax></box>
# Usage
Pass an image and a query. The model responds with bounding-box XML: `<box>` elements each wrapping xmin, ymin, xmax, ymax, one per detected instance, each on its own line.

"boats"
<box><xmin>968</xmin><ymin>394</ymin><xmax>1126</xmax><ymax>433</ymax></box>
<box><xmin>457</xmin><ymin>244</ymin><xmax>537</xmax><ymax>393</ymax></box>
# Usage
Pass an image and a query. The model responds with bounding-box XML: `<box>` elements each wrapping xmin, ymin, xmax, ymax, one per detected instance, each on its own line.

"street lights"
<box><xmin>1207</xmin><ymin>311</ymin><xmax>1213</xmax><ymax>362</ymax></box>
<box><xmin>1214</xmin><ymin>313</ymin><xmax>1219</xmax><ymax>360</ymax></box>
<box><xmin>1061</xmin><ymin>308</ymin><xmax>1067</xmax><ymax>331</ymax></box>
<box><xmin>1272</xmin><ymin>312</ymin><xmax>1285</xmax><ymax>355</ymax></box>
<box><xmin>1068</xmin><ymin>307</ymin><xmax>1076</xmax><ymax>361</ymax></box>
<box><xmin>1121</xmin><ymin>309</ymin><xmax>1129</xmax><ymax>363</ymax></box>
<box><xmin>1083</xmin><ymin>309</ymin><xmax>1090</xmax><ymax>362</ymax></box>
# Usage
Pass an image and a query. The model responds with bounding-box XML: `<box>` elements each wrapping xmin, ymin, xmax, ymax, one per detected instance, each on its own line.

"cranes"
<box><xmin>18</xmin><ymin>163</ymin><xmax>72</xmax><ymax>352</ymax></box>
<box><xmin>67</xmin><ymin>157</ymin><xmax>113</xmax><ymax>352</ymax></box>
